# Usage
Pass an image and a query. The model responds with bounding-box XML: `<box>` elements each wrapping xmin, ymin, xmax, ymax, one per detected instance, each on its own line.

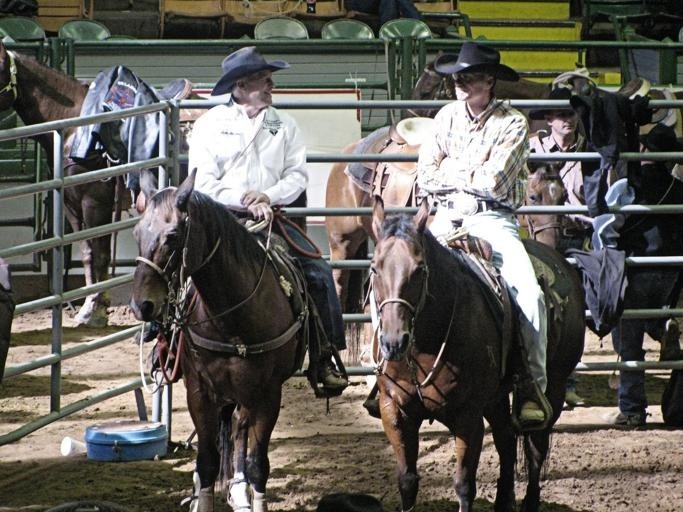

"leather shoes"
<box><xmin>659</xmin><ymin>320</ymin><xmax>681</xmax><ymax>363</ymax></box>
<box><xmin>518</xmin><ymin>399</ymin><xmax>546</xmax><ymax>427</ymax></box>
<box><xmin>314</xmin><ymin>360</ymin><xmax>349</xmax><ymax>388</ymax></box>
<box><xmin>152</xmin><ymin>361</ymin><xmax>172</xmax><ymax>386</ymax></box>
<box><xmin>611</xmin><ymin>411</ymin><xmax>647</xmax><ymax>426</ymax></box>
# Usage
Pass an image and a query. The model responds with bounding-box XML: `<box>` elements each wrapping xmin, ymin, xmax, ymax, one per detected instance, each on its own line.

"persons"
<box><xmin>343</xmin><ymin>0</ymin><xmax>422</xmax><ymax>27</ymax></box>
<box><xmin>147</xmin><ymin>43</ymin><xmax>349</xmax><ymax>389</ymax></box>
<box><xmin>525</xmin><ymin>87</ymin><xmax>601</xmax><ymax>252</ymax></box>
<box><xmin>418</xmin><ymin>43</ymin><xmax>549</xmax><ymax>422</ymax></box>
<box><xmin>599</xmin><ymin>122</ymin><xmax>683</xmax><ymax>427</ymax></box>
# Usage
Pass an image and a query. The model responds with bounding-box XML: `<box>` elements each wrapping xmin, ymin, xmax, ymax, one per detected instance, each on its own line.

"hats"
<box><xmin>211</xmin><ymin>45</ymin><xmax>289</xmax><ymax>95</ymax></box>
<box><xmin>639</xmin><ymin>124</ymin><xmax>682</xmax><ymax>152</ymax></box>
<box><xmin>528</xmin><ymin>87</ymin><xmax>572</xmax><ymax>120</ymax></box>
<box><xmin>434</xmin><ymin>42</ymin><xmax>519</xmax><ymax>83</ymax></box>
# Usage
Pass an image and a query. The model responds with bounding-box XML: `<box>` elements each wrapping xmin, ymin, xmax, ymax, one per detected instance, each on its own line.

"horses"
<box><xmin>0</xmin><ymin>36</ymin><xmax>310</xmax><ymax>334</ymax></box>
<box><xmin>363</xmin><ymin>191</ymin><xmax>590</xmax><ymax>512</ymax></box>
<box><xmin>321</xmin><ymin>120</ymin><xmax>573</xmax><ymax>346</ymax></box>
<box><xmin>129</xmin><ymin>166</ymin><xmax>315</xmax><ymax>511</ymax></box>
<box><xmin>402</xmin><ymin>49</ymin><xmax>683</xmax><ymax>181</ymax></box>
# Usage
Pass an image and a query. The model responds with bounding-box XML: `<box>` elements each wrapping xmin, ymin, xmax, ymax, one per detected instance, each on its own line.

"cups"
<box><xmin>60</xmin><ymin>437</ymin><xmax>86</xmax><ymax>457</ymax></box>
